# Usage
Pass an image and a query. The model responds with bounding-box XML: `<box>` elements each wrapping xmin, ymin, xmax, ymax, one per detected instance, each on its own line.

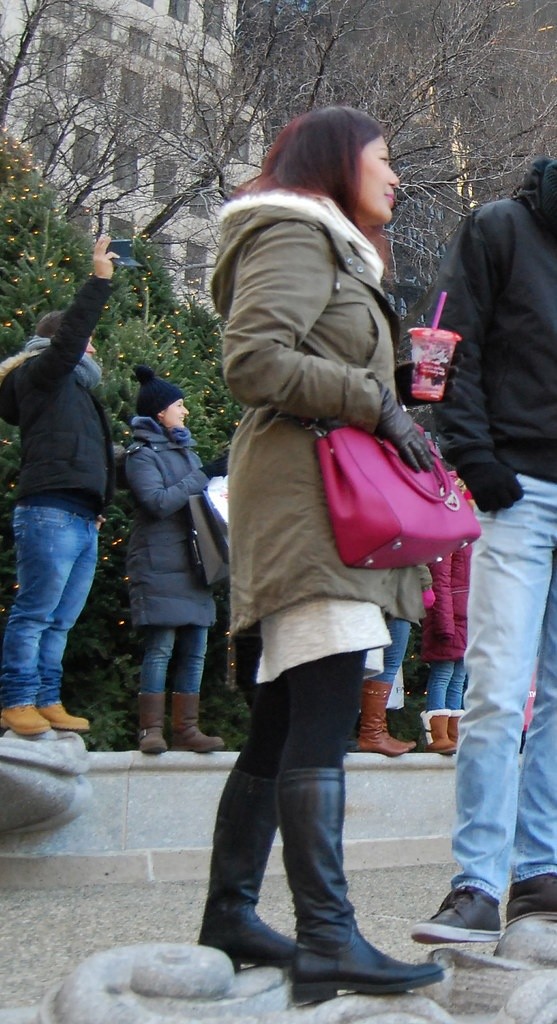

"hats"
<box><xmin>132</xmin><ymin>364</ymin><xmax>184</xmax><ymax>417</ymax></box>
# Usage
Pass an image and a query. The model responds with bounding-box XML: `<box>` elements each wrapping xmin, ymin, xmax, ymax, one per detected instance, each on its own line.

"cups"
<box><xmin>407</xmin><ymin>327</ymin><xmax>461</xmax><ymax>401</ymax></box>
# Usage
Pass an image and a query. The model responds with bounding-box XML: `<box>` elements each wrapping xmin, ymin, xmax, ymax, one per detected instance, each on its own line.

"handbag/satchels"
<box><xmin>187</xmin><ymin>476</ymin><xmax>228</xmax><ymax>587</ymax></box>
<box><xmin>313</xmin><ymin>421</ymin><xmax>482</xmax><ymax>571</ymax></box>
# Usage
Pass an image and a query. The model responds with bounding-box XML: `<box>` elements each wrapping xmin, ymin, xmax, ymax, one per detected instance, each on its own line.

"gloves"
<box><xmin>377</xmin><ymin>389</ymin><xmax>437</xmax><ymax>474</ymax></box>
<box><xmin>421</xmin><ymin>588</ymin><xmax>437</xmax><ymax>608</ymax></box>
<box><xmin>397</xmin><ymin>354</ymin><xmax>463</xmax><ymax>407</ymax></box>
<box><xmin>460</xmin><ymin>461</ymin><xmax>523</xmax><ymax>513</ymax></box>
<box><xmin>200</xmin><ymin>454</ymin><xmax>228</xmax><ymax>480</ymax></box>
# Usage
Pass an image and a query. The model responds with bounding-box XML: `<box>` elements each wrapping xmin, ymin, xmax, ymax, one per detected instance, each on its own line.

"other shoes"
<box><xmin>410</xmin><ymin>888</ymin><xmax>499</xmax><ymax>944</ymax></box>
<box><xmin>36</xmin><ymin>704</ymin><xmax>91</xmax><ymax>731</ymax></box>
<box><xmin>504</xmin><ymin>873</ymin><xmax>557</xmax><ymax>928</ymax></box>
<box><xmin>1</xmin><ymin>706</ymin><xmax>51</xmax><ymax>736</ymax></box>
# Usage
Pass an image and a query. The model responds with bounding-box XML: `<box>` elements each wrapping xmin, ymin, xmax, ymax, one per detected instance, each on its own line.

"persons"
<box><xmin>356</xmin><ymin>563</ymin><xmax>436</xmax><ymax>757</ymax></box>
<box><xmin>198</xmin><ymin>106</ymin><xmax>444</xmax><ymax>1003</ymax></box>
<box><xmin>411</xmin><ymin>157</ymin><xmax>557</xmax><ymax>944</ymax></box>
<box><xmin>125</xmin><ymin>364</ymin><xmax>229</xmax><ymax>753</ymax></box>
<box><xmin>0</xmin><ymin>234</ymin><xmax>120</xmax><ymax>735</ymax></box>
<box><xmin>420</xmin><ymin>471</ymin><xmax>476</xmax><ymax>753</ymax></box>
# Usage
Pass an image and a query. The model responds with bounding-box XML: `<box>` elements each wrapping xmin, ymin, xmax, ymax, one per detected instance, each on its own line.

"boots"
<box><xmin>356</xmin><ymin>679</ymin><xmax>416</xmax><ymax>756</ymax></box>
<box><xmin>275</xmin><ymin>768</ymin><xmax>443</xmax><ymax>1002</ymax></box>
<box><xmin>136</xmin><ymin>692</ymin><xmax>168</xmax><ymax>754</ymax></box>
<box><xmin>197</xmin><ymin>767</ymin><xmax>295</xmax><ymax>967</ymax></box>
<box><xmin>420</xmin><ymin>710</ymin><xmax>465</xmax><ymax>754</ymax></box>
<box><xmin>172</xmin><ymin>691</ymin><xmax>225</xmax><ymax>751</ymax></box>
